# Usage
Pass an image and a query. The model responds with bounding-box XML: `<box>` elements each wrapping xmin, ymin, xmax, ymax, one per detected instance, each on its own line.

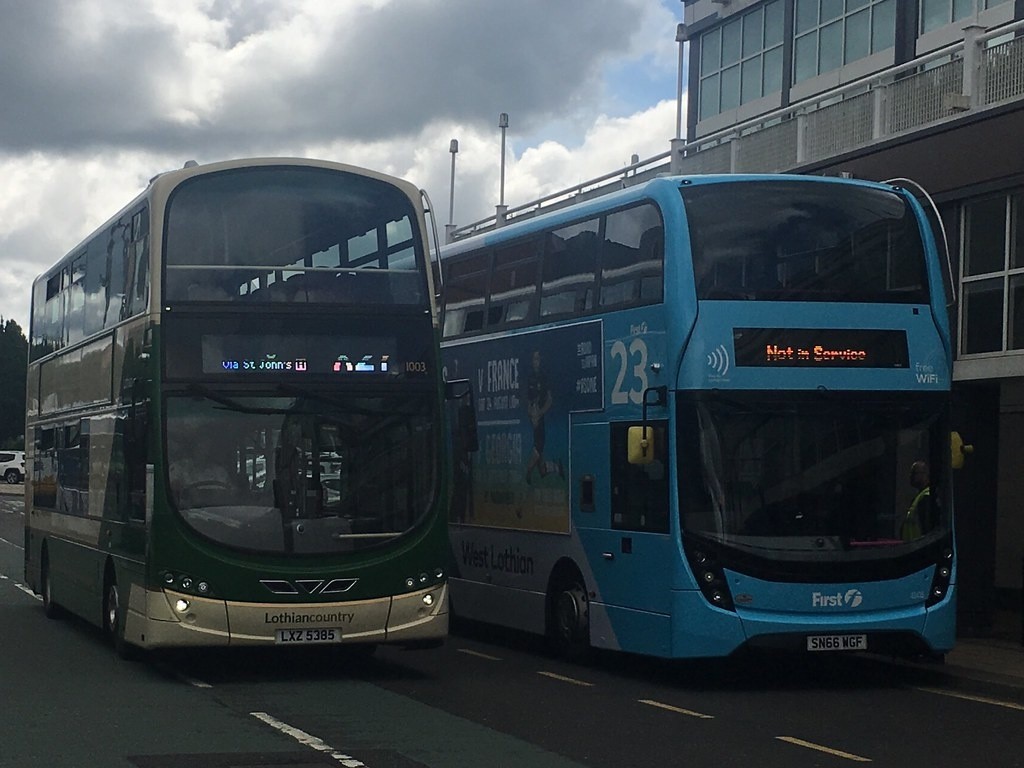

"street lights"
<box><xmin>444</xmin><ymin>140</ymin><xmax>461</xmax><ymax>245</ymax></box>
<box><xmin>496</xmin><ymin>112</ymin><xmax>511</xmax><ymax>227</ymax></box>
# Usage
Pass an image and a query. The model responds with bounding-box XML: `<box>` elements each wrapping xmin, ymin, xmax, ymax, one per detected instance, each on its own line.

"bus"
<box><xmin>386</xmin><ymin>169</ymin><xmax>973</xmax><ymax>678</ymax></box>
<box><xmin>27</xmin><ymin>155</ymin><xmax>485</xmax><ymax>658</ymax></box>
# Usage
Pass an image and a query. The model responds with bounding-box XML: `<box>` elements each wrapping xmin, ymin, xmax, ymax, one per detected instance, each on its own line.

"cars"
<box><xmin>240</xmin><ymin>451</ymin><xmax>341</xmax><ymax>504</ymax></box>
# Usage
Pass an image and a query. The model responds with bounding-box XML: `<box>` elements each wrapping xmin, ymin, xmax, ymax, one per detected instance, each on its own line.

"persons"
<box><xmin>169</xmin><ymin>437</ymin><xmax>231</xmax><ymax>491</ymax></box>
<box><xmin>526</xmin><ymin>347</ymin><xmax>564</xmax><ymax>483</ymax></box>
<box><xmin>900</xmin><ymin>462</ymin><xmax>939</xmax><ymax>542</ymax></box>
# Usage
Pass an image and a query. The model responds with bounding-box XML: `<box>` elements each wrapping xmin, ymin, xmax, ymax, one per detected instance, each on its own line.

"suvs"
<box><xmin>0</xmin><ymin>451</ymin><xmax>26</xmax><ymax>484</ymax></box>
